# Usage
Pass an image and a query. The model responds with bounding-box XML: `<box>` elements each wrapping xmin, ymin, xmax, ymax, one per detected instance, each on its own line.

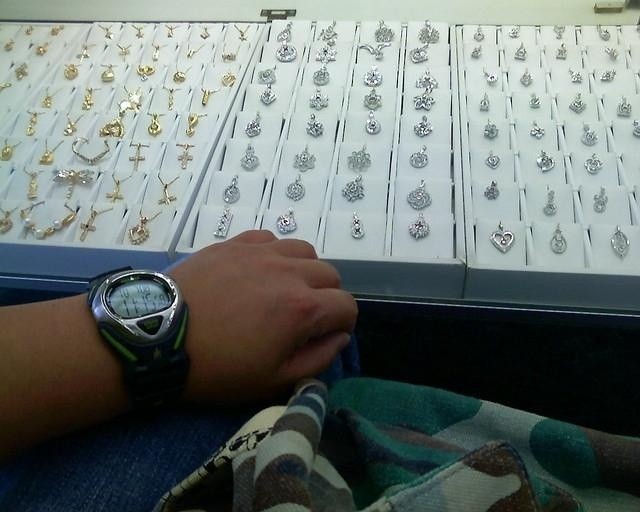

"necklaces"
<box><xmin>40</xmin><ymin>84</ymin><xmax>65</xmax><ymax>109</ymax></box>
<box><xmin>98</xmin><ymin>116</ymin><xmax>124</xmax><ymax>139</ymax></box>
<box><xmin>82</xmin><ymin>87</ymin><xmax>101</xmax><ymax>111</ymax></box>
<box><xmin>22</xmin><ymin>167</ymin><xmax>44</xmax><ymax>199</ymax></box>
<box><xmin>163</xmin><ymin>85</ymin><xmax>182</xmax><ymax>111</ymax></box>
<box><xmin>77</xmin><ymin>42</ymin><xmax>97</xmax><ymax>63</ymax></box>
<box><xmin>0</xmin><ymin>82</ymin><xmax>12</xmax><ymax>94</ymax></box>
<box><xmin>54</xmin><ymin>168</ymin><xmax>94</xmax><ymax>199</ymax></box>
<box><xmin>0</xmin><ymin>206</ymin><xmax>19</xmax><ymax>234</ymax></box>
<box><xmin>0</xmin><ymin>139</ymin><xmax>22</xmax><ymax>161</ymax></box>
<box><xmin>24</xmin><ymin>109</ymin><xmax>46</xmax><ymax>137</ymax></box>
<box><xmin>127</xmin><ymin>207</ymin><xmax>163</xmax><ymax>246</ymax></box>
<box><xmin>156</xmin><ymin>173</ymin><xmax>179</xmax><ymax>205</ymax></box>
<box><xmin>128</xmin><ymin>142</ymin><xmax>149</xmax><ymax>171</ymax></box>
<box><xmin>39</xmin><ymin>138</ymin><xmax>64</xmax><ymax>165</ymax></box>
<box><xmin>62</xmin><ymin>112</ymin><xmax>85</xmax><ymax>137</ymax></box>
<box><xmin>4</xmin><ymin>38</ymin><xmax>16</xmax><ymax>52</ymax></box>
<box><xmin>130</xmin><ymin>22</ymin><xmax>149</xmax><ymax>39</ymax></box>
<box><xmin>146</xmin><ymin>112</ymin><xmax>165</xmax><ymax>136</ymax></box>
<box><xmin>152</xmin><ymin>42</ymin><xmax>168</xmax><ymax>61</ymax></box>
<box><xmin>105</xmin><ymin>173</ymin><xmax>133</xmax><ymax>203</ymax></box>
<box><xmin>116</xmin><ymin>42</ymin><xmax>132</xmax><ymax>62</ymax></box>
<box><xmin>101</xmin><ymin>63</ymin><xmax>118</xmax><ymax>83</ymax></box>
<box><xmin>163</xmin><ymin>24</ymin><xmax>183</xmax><ymax>38</ymax></box>
<box><xmin>62</xmin><ymin>59</ymin><xmax>82</xmax><ymax>81</ymax></box>
<box><xmin>33</xmin><ymin>41</ymin><xmax>50</xmax><ymax>55</ymax></box>
<box><xmin>79</xmin><ymin>203</ymin><xmax>114</xmax><ymax>242</ymax></box>
<box><xmin>186</xmin><ymin>41</ymin><xmax>206</xmax><ymax>58</ymax></box>
<box><xmin>173</xmin><ymin>64</ymin><xmax>192</xmax><ymax>83</ymax></box>
<box><xmin>14</xmin><ymin>62</ymin><xmax>29</xmax><ymax>80</ymax></box>
<box><xmin>200</xmin><ymin>26</ymin><xmax>212</xmax><ymax>40</ymax></box>
<box><xmin>200</xmin><ymin>88</ymin><xmax>221</xmax><ymax>107</ymax></box>
<box><xmin>51</xmin><ymin>22</ymin><xmax>64</xmax><ymax>36</ymax></box>
<box><xmin>71</xmin><ymin>137</ymin><xmax>110</xmax><ymax>166</ymax></box>
<box><xmin>185</xmin><ymin>111</ymin><xmax>208</xmax><ymax>138</ymax></box>
<box><xmin>220</xmin><ymin>70</ymin><xmax>235</xmax><ymax>86</ymax></box>
<box><xmin>95</xmin><ymin>21</ymin><xmax>115</xmax><ymax>38</ymax></box>
<box><xmin>135</xmin><ymin>65</ymin><xmax>156</xmax><ymax>83</ymax></box>
<box><xmin>176</xmin><ymin>144</ymin><xmax>195</xmax><ymax>170</ymax></box>
<box><xmin>26</xmin><ymin>25</ymin><xmax>35</xmax><ymax>35</ymax></box>
<box><xmin>221</xmin><ymin>41</ymin><xmax>240</xmax><ymax>62</ymax></box>
<box><xmin>21</xmin><ymin>201</ymin><xmax>78</xmax><ymax>239</ymax></box>
<box><xmin>117</xmin><ymin>83</ymin><xmax>141</xmax><ymax>116</ymax></box>
<box><xmin>234</xmin><ymin>23</ymin><xmax>251</xmax><ymax>41</ymax></box>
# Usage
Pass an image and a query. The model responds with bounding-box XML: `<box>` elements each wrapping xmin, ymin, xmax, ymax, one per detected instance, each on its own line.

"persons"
<box><xmin>1</xmin><ymin>229</ymin><xmax>359</xmax><ymax>512</ymax></box>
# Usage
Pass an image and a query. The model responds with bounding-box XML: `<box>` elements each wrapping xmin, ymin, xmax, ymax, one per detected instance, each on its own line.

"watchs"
<box><xmin>87</xmin><ymin>265</ymin><xmax>192</xmax><ymax>412</ymax></box>
